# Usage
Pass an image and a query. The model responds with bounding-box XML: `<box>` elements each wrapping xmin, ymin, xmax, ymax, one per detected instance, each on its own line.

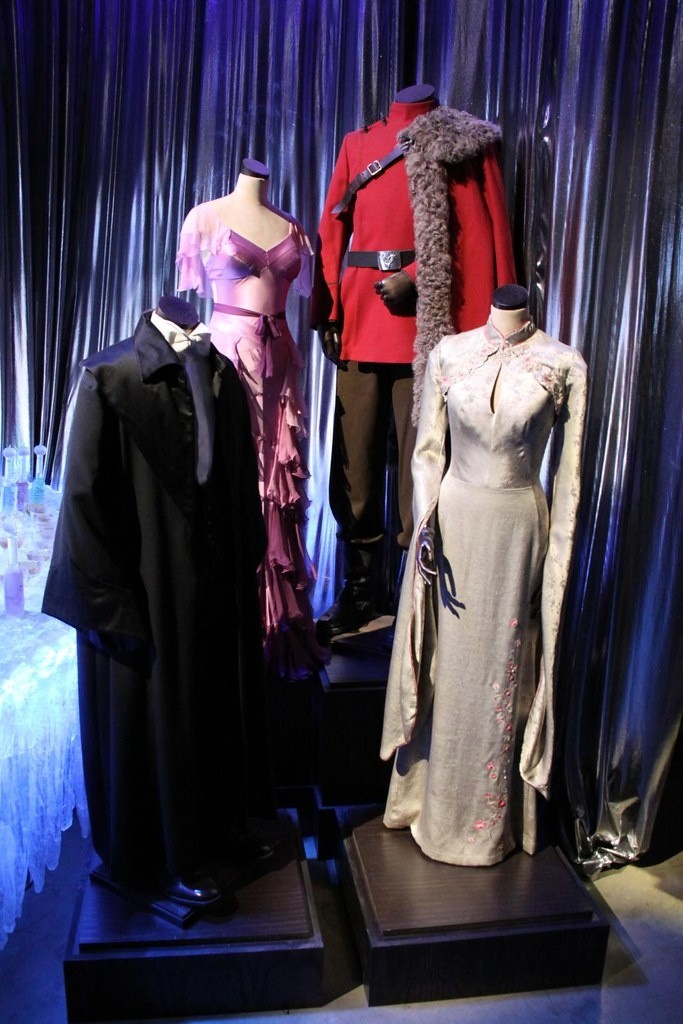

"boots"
<box><xmin>316</xmin><ymin>534</ymin><xmax>391</xmax><ymax>636</ymax></box>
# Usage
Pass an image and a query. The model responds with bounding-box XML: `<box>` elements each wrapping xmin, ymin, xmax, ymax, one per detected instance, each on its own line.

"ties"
<box><xmin>182</xmin><ymin>348</ymin><xmax>211</xmax><ymax>486</ymax></box>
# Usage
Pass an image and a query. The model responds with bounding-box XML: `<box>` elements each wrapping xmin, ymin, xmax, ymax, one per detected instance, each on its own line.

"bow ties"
<box><xmin>172</xmin><ymin>333</ymin><xmax>210</xmax><ymax>357</ymax></box>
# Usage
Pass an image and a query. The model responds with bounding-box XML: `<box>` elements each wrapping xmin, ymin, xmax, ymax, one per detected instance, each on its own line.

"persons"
<box><xmin>44</xmin><ymin>298</ymin><xmax>269</xmax><ymax>906</ymax></box>
<box><xmin>307</xmin><ymin>82</ymin><xmax>519</xmax><ymax>635</ymax></box>
<box><xmin>378</xmin><ymin>282</ymin><xmax>588</xmax><ymax>867</ymax></box>
<box><xmin>169</xmin><ymin>157</ymin><xmax>321</xmax><ymax>691</ymax></box>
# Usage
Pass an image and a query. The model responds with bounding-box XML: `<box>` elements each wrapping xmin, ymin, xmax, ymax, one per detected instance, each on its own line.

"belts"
<box><xmin>347</xmin><ymin>250</ymin><xmax>416</xmax><ymax>271</ymax></box>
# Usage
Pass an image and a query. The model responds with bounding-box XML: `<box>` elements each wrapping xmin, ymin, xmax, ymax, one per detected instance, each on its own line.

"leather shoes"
<box><xmin>161</xmin><ymin>871</ymin><xmax>221</xmax><ymax>906</ymax></box>
<box><xmin>244</xmin><ymin>845</ymin><xmax>274</xmax><ymax>860</ymax></box>
